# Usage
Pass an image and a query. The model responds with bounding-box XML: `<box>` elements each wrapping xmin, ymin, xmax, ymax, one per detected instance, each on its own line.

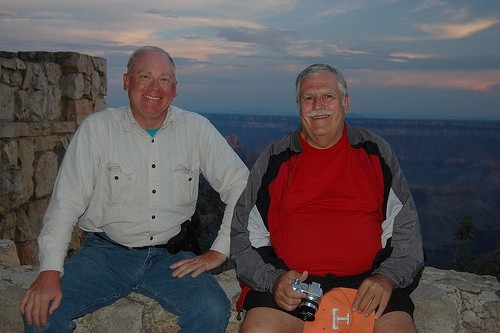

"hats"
<box><xmin>304</xmin><ymin>288</ymin><xmax>375</xmax><ymax>333</ymax></box>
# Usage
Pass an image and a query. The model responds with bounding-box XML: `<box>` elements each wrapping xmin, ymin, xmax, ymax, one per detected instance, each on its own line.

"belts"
<box><xmin>94</xmin><ymin>232</ymin><xmax>168</xmax><ymax>250</ymax></box>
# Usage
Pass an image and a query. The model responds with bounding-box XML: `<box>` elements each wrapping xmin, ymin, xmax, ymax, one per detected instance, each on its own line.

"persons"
<box><xmin>19</xmin><ymin>45</ymin><xmax>250</xmax><ymax>332</ymax></box>
<box><xmin>230</xmin><ymin>62</ymin><xmax>425</xmax><ymax>333</ymax></box>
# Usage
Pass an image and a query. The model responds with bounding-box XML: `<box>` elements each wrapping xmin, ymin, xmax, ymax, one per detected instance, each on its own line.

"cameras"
<box><xmin>291</xmin><ymin>279</ymin><xmax>322</xmax><ymax>321</ymax></box>
